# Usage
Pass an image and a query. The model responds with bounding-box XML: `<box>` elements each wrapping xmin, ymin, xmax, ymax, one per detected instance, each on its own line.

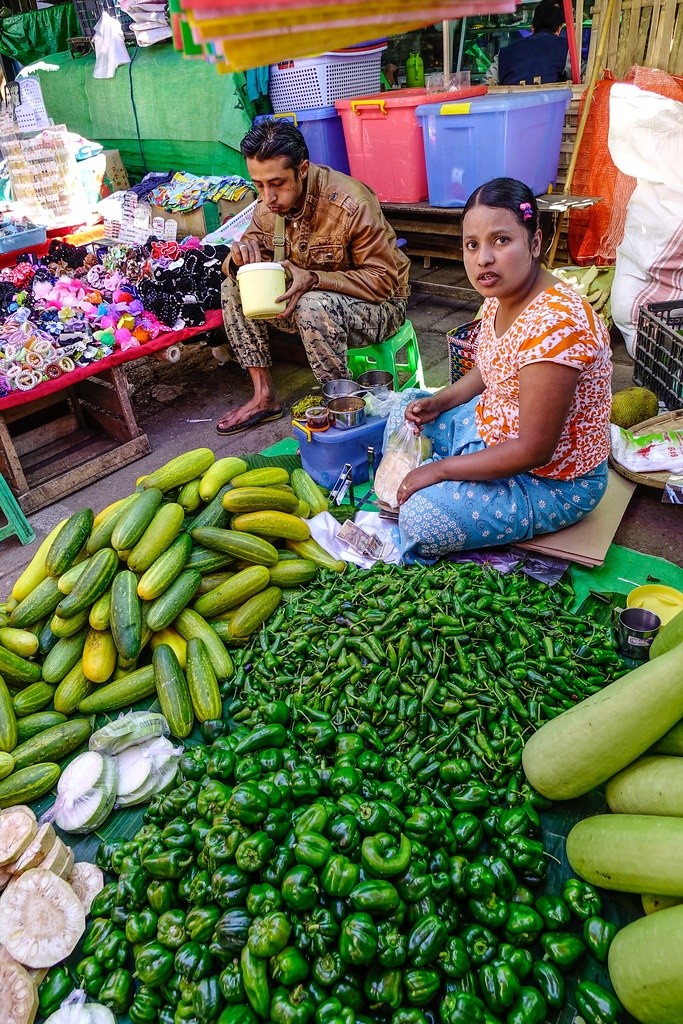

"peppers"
<box><xmin>35</xmin><ymin>559</ymin><xmax>651</xmax><ymax>1024</ymax></box>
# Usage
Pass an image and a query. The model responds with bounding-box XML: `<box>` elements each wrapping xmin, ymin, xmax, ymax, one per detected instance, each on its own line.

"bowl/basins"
<box><xmin>357</xmin><ymin>370</ymin><xmax>394</xmax><ymax>396</ymax></box>
<box><xmin>327</xmin><ymin>396</ymin><xmax>366</xmax><ymax>430</ymax></box>
<box><xmin>322</xmin><ymin>378</ymin><xmax>361</xmax><ymax>408</ymax></box>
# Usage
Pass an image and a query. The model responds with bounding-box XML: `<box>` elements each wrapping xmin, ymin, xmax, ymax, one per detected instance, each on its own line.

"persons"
<box><xmin>380</xmin><ymin>59</ymin><xmax>399</xmax><ymax>92</ymax></box>
<box><xmin>480</xmin><ymin>0</ymin><xmax>586</xmax><ymax>86</ymax></box>
<box><xmin>381</xmin><ymin>177</ymin><xmax>613</xmax><ymax>564</ymax></box>
<box><xmin>216</xmin><ymin>117</ymin><xmax>411</xmax><ymax>435</ymax></box>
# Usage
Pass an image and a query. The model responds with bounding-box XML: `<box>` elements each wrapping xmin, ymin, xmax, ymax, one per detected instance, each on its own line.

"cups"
<box><xmin>236</xmin><ymin>262</ymin><xmax>287</xmax><ymax>320</ymax></box>
<box><xmin>305</xmin><ymin>407</ymin><xmax>330</xmax><ymax>432</ymax></box>
<box><xmin>611</xmin><ymin>607</ymin><xmax>662</xmax><ymax>660</ymax></box>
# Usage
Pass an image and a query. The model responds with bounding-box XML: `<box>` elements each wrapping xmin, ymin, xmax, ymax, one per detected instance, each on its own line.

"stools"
<box><xmin>346</xmin><ymin>319</ymin><xmax>425</xmax><ymax>393</ymax></box>
<box><xmin>0</xmin><ymin>472</ymin><xmax>37</xmax><ymax>546</ymax></box>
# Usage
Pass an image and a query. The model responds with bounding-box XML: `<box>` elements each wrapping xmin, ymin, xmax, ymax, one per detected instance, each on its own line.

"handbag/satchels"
<box><xmin>374</xmin><ymin>420</ymin><xmax>421</xmax><ymax>508</ymax></box>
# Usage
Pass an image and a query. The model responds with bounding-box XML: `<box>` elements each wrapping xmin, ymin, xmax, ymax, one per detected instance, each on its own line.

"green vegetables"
<box><xmin>290</xmin><ymin>396</ymin><xmax>322</xmax><ymax>416</ymax></box>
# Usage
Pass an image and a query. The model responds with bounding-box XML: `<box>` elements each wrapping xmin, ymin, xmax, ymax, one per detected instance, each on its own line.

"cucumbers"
<box><xmin>0</xmin><ymin>448</ymin><xmax>345</xmax><ymax>808</ymax></box>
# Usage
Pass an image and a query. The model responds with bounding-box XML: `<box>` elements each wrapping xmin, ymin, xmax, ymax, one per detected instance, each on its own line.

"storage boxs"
<box><xmin>73</xmin><ymin>0</ymin><xmax>135</xmax><ymax>37</ymax></box>
<box><xmin>252</xmin><ymin>36</ymin><xmax>572</xmax><ymax>206</ymax></box>
<box><xmin>150</xmin><ymin>190</ymin><xmax>255</xmax><ymax>237</ymax></box>
<box><xmin>77</xmin><ymin>149</ymin><xmax>131</xmax><ymax>205</ymax></box>
<box><xmin>632</xmin><ymin>299</ymin><xmax>683</xmax><ymax>412</ymax></box>
<box><xmin>290</xmin><ymin>398</ymin><xmax>390</xmax><ymax>491</ymax></box>
<box><xmin>0</xmin><ymin>222</ymin><xmax>47</xmax><ymax>254</ymax></box>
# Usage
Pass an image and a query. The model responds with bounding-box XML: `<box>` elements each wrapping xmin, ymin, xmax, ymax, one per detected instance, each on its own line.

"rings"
<box><xmin>240</xmin><ymin>245</ymin><xmax>247</xmax><ymax>250</ymax></box>
<box><xmin>402</xmin><ymin>485</ymin><xmax>408</xmax><ymax>492</ymax></box>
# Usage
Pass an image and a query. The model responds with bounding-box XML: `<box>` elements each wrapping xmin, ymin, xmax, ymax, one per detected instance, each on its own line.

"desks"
<box><xmin>380</xmin><ymin>193</ymin><xmax>603</xmax><ymax>269</ymax></box>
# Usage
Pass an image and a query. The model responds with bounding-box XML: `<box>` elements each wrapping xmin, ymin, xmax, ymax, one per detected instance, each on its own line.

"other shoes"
<box><xmin>216</xmin><ymin>406</ymin><xmax>283</xmax><ymax>435</ymax></box>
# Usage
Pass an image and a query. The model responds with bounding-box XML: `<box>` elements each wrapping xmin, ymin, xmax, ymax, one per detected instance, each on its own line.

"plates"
<box><xmin>289</xmin><ymin>396</ymin><xmax>322</xmax><ymax>421</ymax></box>
<box><xmin>626</xmin><ymin>584</ymin><xmax>683</xmax><ymax>626</ymax></box>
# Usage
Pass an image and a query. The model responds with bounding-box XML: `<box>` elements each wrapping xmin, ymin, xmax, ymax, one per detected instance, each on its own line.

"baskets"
<box><xmin>269</xmin><ymin>36</ymin><xmax>388</xmax><ymax>114</ymax></box>
